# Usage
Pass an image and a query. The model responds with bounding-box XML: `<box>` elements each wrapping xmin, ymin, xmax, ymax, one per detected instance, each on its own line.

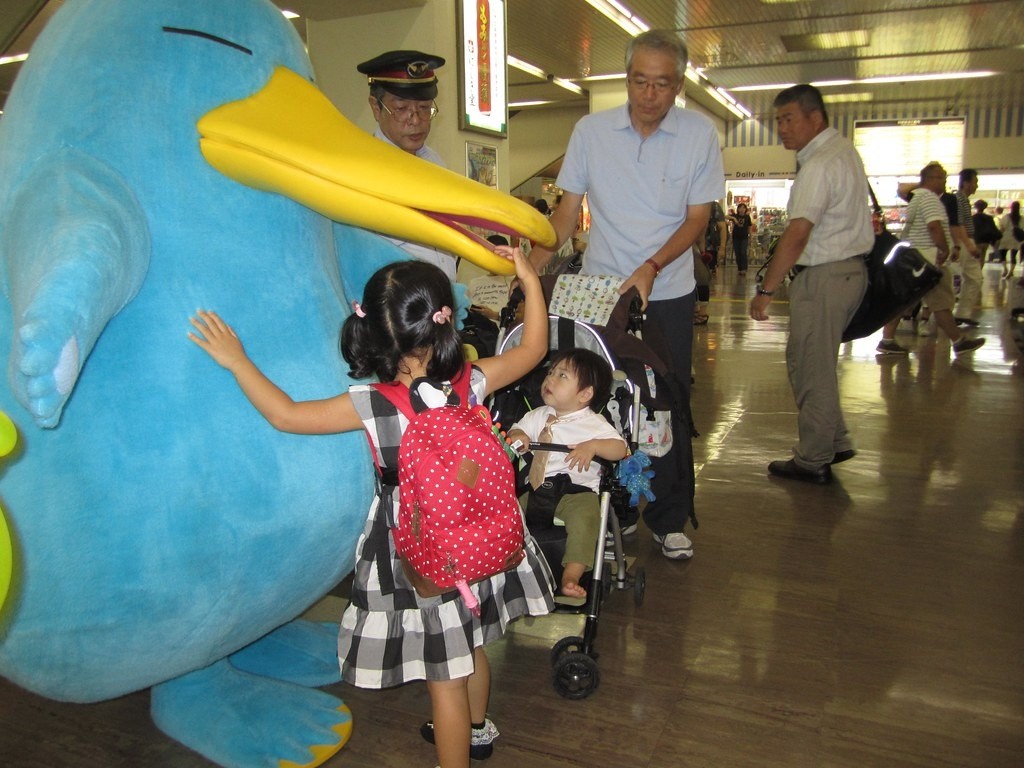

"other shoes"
<box><xmin>419</xmin><ymin>719</ymin><xmax>499</xmax><ymax>761</ymax></box>
<box><xmin>1000</xmin><ymin>271</ymin><xmax>1014</xmax><ymax>280</ymax></box>
<box><xmin>737</xmin><ymin>270</ymin><xmax>746</xmax><ymax>275</ymax></box>
<box><xmin>910</xmin><ymin>318</ymin><xmax>931</xmax><ymax>337</ymax></box>
<box><xmin>1016</xmin><ymin>277</ymin><xmax>1024</xmax><ymax>288</ymax></box>
<box><xmin>953</xmin><ymin>315</ymin><xmax>981</xmax><ymax>326</ymax></box>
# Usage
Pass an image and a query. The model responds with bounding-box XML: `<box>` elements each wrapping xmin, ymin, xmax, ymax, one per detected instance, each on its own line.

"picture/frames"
<box><xmin>455</xmin><ymin>0</ymin><xmax>508</xmax><ymax>138</ymax></box>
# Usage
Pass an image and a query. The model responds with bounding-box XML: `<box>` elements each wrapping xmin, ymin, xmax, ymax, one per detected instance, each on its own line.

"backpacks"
<box><xmin>362</xmin><ymin>359</ymin><xmax>526</xmax><ymax>619</ymax></box>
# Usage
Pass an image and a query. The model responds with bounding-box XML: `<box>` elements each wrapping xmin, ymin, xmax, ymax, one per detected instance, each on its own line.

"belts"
<box><xmin>794</xmin><ymin>264</ymin><xmax>807</xmax><ymax>273</ymax></box>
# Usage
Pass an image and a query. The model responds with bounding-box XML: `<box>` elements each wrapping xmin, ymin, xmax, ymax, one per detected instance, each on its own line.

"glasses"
<box><xmin>625</xmin><ymin>74</ymin><xmax>680</xmax><ymax>92</ymax></box>
<box><xmin>377</xmin><ymin>96</ymin><xmax>439</xmax><ymax>123</ymax></box>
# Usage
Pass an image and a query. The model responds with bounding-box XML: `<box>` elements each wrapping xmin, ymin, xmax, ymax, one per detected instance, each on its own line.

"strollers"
<box><xmin>497</xmin><ymin>277</ymin><xmax>662</xmax><ymax>704</ymax></box>
<box><xmin>753</xmin><ymin>236</ymin><xmax>800</xmax><ymax>285</ymax></box>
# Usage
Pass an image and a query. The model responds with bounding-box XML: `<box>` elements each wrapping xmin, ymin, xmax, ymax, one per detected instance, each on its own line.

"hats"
<box><xmin>356</xmin><ymin>49</ymin><xmax>446</xmax><ymax>101</ymax></box>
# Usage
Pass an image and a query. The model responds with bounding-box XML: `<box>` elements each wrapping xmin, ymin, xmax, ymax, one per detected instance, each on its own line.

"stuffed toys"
<box><xmin>0</xmin><ymin>1</ymin><xmax>556</xmax><ymax>768</ymax></box>
<box><xmin>617</xmin><ymin>451</ymin><xmax>656</xmax><ymax>505</ymax></box>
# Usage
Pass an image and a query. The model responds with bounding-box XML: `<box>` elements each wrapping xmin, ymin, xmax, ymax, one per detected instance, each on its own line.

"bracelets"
<box><xmin>646</xmin><ymin>258</ymin><xmax>662</xmax><ymax>276</ymax></box>
<box><xmin>756</xmin><ymin>285</ymin><xmax>775</xmax><ymax>297</ymax></box>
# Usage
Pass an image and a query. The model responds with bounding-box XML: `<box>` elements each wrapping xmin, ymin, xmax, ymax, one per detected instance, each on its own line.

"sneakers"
<box><xmin>952</xmin><ymin>335</ymin><xmax>986</xmax><ymax>356</ymax></box>
<box><xmin>652</xmin><ymin>531</ymin><xmax>694</xmax><ymax>560</ymax></box>
<box><xmin>604</xmin><ymin>523</ymin><xmax>638</xmax><ymax>547</ymax></box>
<box><xmin>875</xmin><ymin>340</ymin><xmax>909</xmax><ymax>355</ymax></box>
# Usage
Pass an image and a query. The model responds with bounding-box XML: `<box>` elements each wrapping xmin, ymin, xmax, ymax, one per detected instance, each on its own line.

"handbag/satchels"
<box><xmin>628</xmin><ymin>398</ymin><xmax>673</xmax><ymax>458</ymax></box>
<box><xmin>1011</xmin><ymin>227</ymin><xmax>1024</xmax><ymax>242</ymax></box>
<box><xmin>841</xmin><ymin>229</ymin><xmax>945</xmax><ymax>344</ymax></box>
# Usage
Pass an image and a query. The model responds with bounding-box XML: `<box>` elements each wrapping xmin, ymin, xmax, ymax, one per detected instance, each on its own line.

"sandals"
<box><xmin>692</xmin><ymin>311</ymin><xmax>710</xmax><ymax>326</ymax></box>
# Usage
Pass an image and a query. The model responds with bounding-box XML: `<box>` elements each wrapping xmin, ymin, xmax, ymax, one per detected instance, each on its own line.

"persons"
<box><xmin>750</xmin><ymin>84</ymin><xmax>875</xmax><ymax>482</ymax></box>
<box><xmin>354</xmin><ymin>50</ymin><xmax>448</xmax><ymax>167</ymax></box>
<box><xmin>187</xmin><ymin>246</ymin><xmax>548</xmax><ymax>767</ymax></box>
<box><xmin>534</xmin><ymin>195</ymin><xmax>572</xmax><ymax>258</ymax></box>
<box><xmin>992</xmin><ymin>201</ymin><xmax>1024</xmax><ymax>285</ymax></box>
<box><xmin>468</xmin><ymin>235</ymin><xmax>520</xmax><ymax>363</ymax></box>
<box><xmin>693</xmin><ymin>202</ymin><xmax>751</xmax><ymax>323</ymax></box>
<box><xmin>506</xmin><ymin>349</ymin><xmax>627</xmax><ymax>600</ymax></box>
<box><xmin>510</xmin><ymin>30</ymin><xmax>726</xmax><ymax>563</ymax></box>
<box><xmin>876</xmin><ymin>162</ymin><xmax>997</xmax><ymax>359</ymax></box>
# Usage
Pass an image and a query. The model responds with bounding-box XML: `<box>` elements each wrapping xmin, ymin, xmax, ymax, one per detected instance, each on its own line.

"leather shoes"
<box><xmin>766</xmin><ymin>458</ymin><xmax>833</xmax><ymax>486</ymax></box>
<box><xmin>830</xmin><ymin>449</ymin><xmax>857</xmax><ymax>466</ymax></box>
